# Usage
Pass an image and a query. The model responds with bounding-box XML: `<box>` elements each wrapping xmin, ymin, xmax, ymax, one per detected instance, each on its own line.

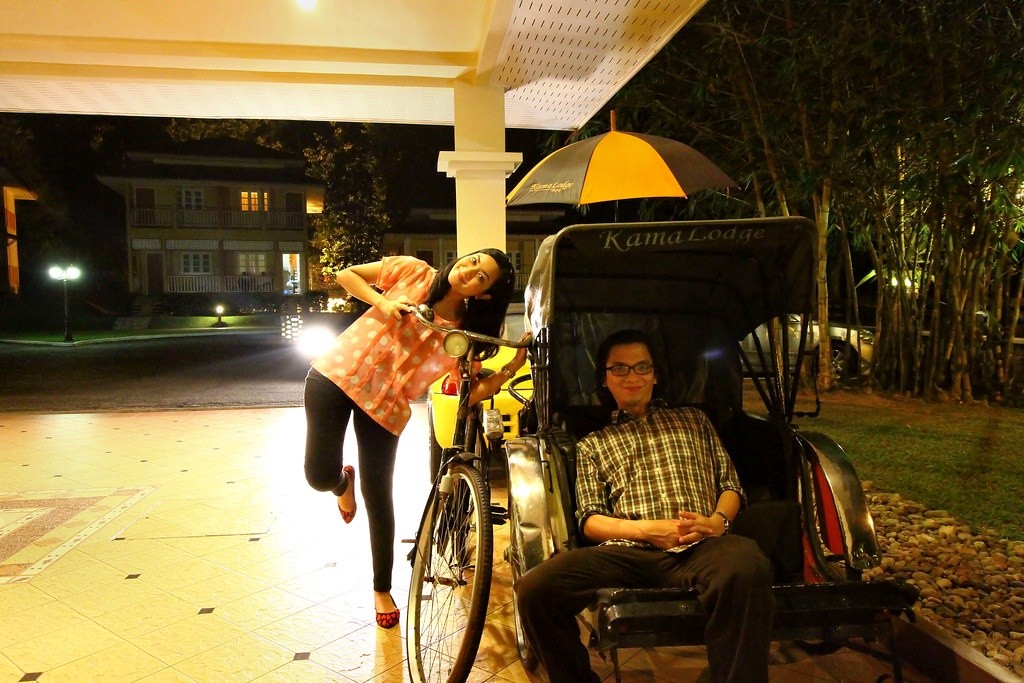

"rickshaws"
<box><xmin>503</xmin><ymin>216</ymin><xmax>917</xmax><ymax>682</ymax></box>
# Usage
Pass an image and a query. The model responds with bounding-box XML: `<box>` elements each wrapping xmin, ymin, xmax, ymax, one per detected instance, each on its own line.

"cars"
<box><xmin>740</xmin><ymin>313</ymin><xmax>876</xmax><ymax>389</ymax></box>
<box><xmin>425</xmin><ymin>320</ymin><xmax>540</xmax><ymax>488</ymax></box>
<box><xmin>812</xmin><ymin>277</ymin><xmax>985</xmax><ymax>333</ymax></box>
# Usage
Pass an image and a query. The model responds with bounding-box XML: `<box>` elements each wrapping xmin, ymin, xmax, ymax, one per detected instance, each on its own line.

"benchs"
<box><xmin>552</xmin><ymin>399</ymin><xmax>803</xmax><ymax>583</ymax></box>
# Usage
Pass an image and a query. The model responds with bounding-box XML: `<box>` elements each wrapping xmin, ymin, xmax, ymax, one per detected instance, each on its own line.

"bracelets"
<box><xmin>501</xmin><ymin>365</ymin><xmax>516</xmax><ymax>378</ymax></box>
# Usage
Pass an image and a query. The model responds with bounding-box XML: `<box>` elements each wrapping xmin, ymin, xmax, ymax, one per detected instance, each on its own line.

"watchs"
<box><xmin>713</xmin><ymin>512</ymin><xmax>731</xmax><ymax>534</ymax></box>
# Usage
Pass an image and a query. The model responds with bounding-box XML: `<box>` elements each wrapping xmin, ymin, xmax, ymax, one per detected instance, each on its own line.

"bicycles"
<box><xmin>397</xmin><ymin>303</ymin><xmax>535</xmax><ymax>683</ymax></box>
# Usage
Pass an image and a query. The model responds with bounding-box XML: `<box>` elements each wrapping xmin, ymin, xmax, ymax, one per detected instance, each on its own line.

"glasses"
<box><xmin>606</xmin><ymin>361</ymin><xmax>653</xmax><ymax>375</ymax></box>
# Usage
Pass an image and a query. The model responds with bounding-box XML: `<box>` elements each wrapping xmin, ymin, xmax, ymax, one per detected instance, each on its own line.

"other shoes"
<box><xmin>375</xmin><ymin>594</ymin><xmax>400</xmax><ymax>629</ymax></box>
<box><xmin>338</xmin><ymin>465</ymin><xmax>356</xmax><ymax>524</ymax></box>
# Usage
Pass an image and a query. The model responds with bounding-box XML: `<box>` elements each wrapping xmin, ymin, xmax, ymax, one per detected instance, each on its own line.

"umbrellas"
<box><xmin>505</xmin><ymin>110</ymin><xmax>742</xmax><ymax>223</ymax></box>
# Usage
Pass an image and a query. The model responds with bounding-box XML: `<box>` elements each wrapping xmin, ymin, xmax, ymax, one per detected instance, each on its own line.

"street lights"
<box><xmin>49</xmin><ymin>264</ymin><xmax>81</xmax><ymax>343</ymax></box>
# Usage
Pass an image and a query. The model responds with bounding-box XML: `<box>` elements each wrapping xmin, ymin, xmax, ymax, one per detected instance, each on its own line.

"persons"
<box><xmin>515</xmin><ymin>329</ymin><xmax>772</xmax><ymax>683</ymax></box>
<box><xmin>306</xmin><ymin>248</ymin><xmax>516</xmax><ymax>628</ymax></box>
